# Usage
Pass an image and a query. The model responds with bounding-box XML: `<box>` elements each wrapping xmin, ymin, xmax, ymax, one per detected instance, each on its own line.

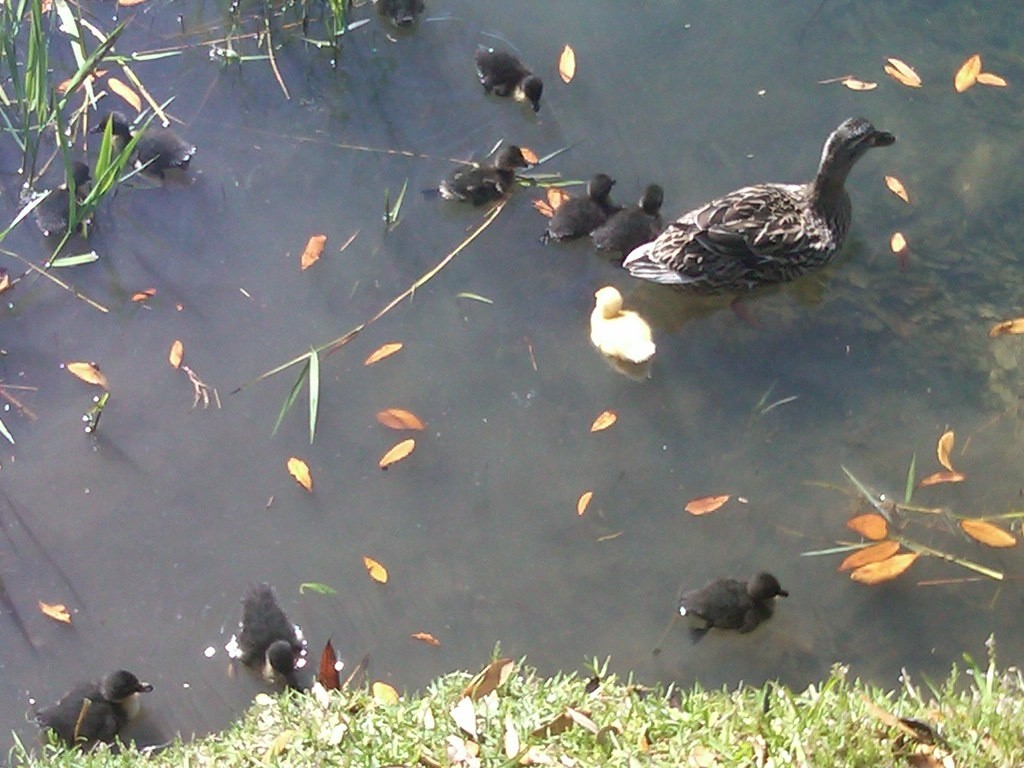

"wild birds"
<box><xmin>89</xmin><ymin>110</ymin><xmax>197</xmax><ymax>187</ymax></box>
<box><xmin>444</xmin><ymin>144</ymin><xmax>529</xmax><ymax>206</ymax></box>
<box><xmin>591</xmin><ymin>285</ymin><xmax>654</xmax><ymax>362</ymax></box>
<box><xmin>376</xmin><ymin>0</ymin><xmax>425</xmax><ymax>27</ymax></box>
<box><xmin>238</xmin><ymin>579</ymin><xmax>306</xmax><ymax>691</ymax></box>
<box><xmin>33</xmin><ymin>161</ymin><xmax>94</xmax><ymax>235</ymax></box>
<box><xmin>542</xmin><ymin>173</ymin><xmax>617</xmax><ymax>244</ymax></box>
<box><xmin>621</xmin><ymin>116</ymin><xmax>896</xmax><ymax>295</ymax></box>
<box><xmin>682</xmin><ymin>573</ymin><xmax>790</xmax><ymax>634</ymax></box>
<box><xmin>589</xmin><ymin>182</ymin><xmax>663</xmax><ymax>252</ymax></box>
<box><xmin>32</xmin><ymin>670</ymin><xmax>156</xmax><ymax>747</ymax></box>
<box><xmin>473</xmin><ymin>45</ymin><xmax>543</xmax><ymax>115</ymax></box>
